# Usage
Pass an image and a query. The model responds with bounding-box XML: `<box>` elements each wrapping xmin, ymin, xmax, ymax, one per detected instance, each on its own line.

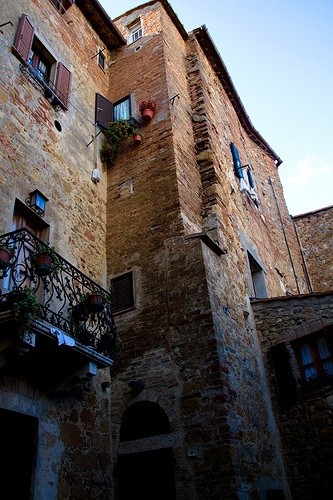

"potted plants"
<box><xmin>131</xmin><ymin>129</ymin><xmax>145</xmax><ymax>142</ymax></box>
<box><xmin>79</xmin><ymin>290</ymin><xmax>111</xmax><ymax>312</ymax></box>
<box><xmin>30</xmin><ymin>242</ymin><xmax>63</xmax><ymax>276</ymax></box>
<box><xmin>0</xmin><ymin>242</ymin><xmax>15</xmax><ymax>268</ymax></box>
<box><xmin>139</xmin><ymin>98</ymin><xmax>157</xmax><ymax>119</ymax></box>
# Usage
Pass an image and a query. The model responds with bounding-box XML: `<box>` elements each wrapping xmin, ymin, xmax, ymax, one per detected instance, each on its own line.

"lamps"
<box><xmin>128</xmin><ymin>378</ymin><xmax>145</xmax><ymax>394</ymax></box>
